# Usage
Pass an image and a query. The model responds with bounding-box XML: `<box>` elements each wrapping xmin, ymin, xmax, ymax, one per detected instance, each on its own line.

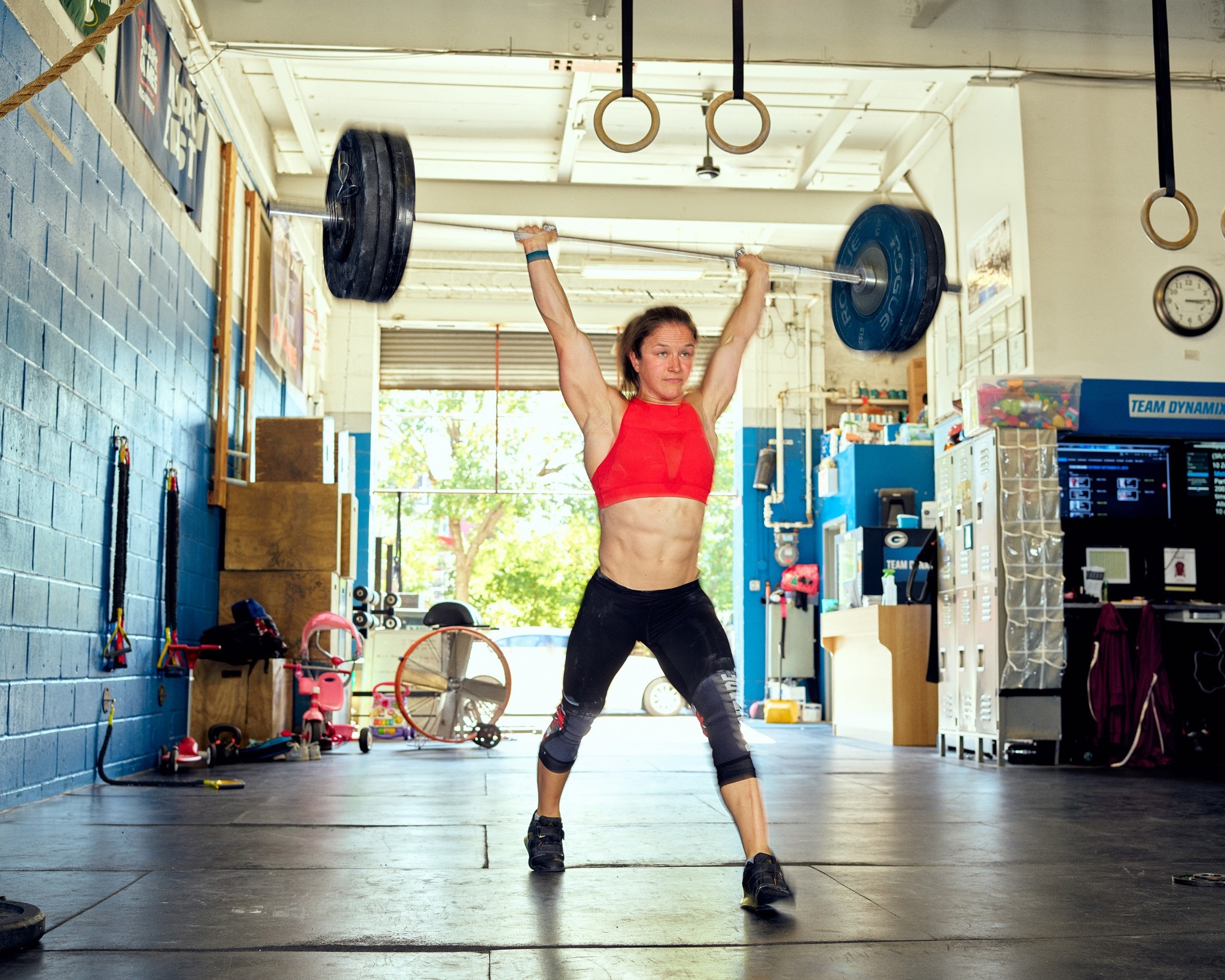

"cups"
<box><xmin>752</xmin><ymin>448</ymin><xmax>776</xmax><ymax>488</ymax></box>
<box><xmin>822</xmin><ymin>598</ymin><xmax>839</xmax><ymax>613</ymax></box>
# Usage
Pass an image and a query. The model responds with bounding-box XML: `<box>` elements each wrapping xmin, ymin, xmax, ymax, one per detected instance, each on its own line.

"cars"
<box><xmin>482</xmin><ymin>625</ymin><xmax>694</xmax><ymax>719</ymax></box>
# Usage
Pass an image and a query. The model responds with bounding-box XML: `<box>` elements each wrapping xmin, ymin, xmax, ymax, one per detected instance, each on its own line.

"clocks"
<box><xmin>1153</xmin><ymin>266</ymin><xmax>1223</xmax><ymax>336</ymax></box>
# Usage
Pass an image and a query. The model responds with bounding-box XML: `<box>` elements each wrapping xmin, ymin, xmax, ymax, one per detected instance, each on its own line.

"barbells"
<box><xmin>261</xmin><ymin>126</ymin><xmax>965</xmax><ymax>359</ymax></box>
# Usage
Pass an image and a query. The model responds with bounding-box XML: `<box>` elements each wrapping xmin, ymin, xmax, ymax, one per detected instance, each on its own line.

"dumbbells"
<box><xmin>351</xmin><ymin>585</ymin><xmax>402</xmax><ymax>630</ymax></box>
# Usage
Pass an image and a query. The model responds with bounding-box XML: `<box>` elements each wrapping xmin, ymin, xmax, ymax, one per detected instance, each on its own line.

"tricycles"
<box><xmin>280</xmin><ymin>611</ymin><xmax>377</xmax><ymax>753</ymax></box>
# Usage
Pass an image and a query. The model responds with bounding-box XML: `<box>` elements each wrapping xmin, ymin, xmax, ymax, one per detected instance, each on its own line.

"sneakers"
<box><xmin>523</xmin><ymin>810</ymin><xmax>565</xmax><ymax>872</ymax></box>
<box><xmin>738</xmin><ymin>851</ymin><xmax>793</xmax><ymax>910</ymax></box>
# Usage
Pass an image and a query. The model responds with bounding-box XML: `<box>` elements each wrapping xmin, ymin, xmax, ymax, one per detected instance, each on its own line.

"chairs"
<box><xmin>313</xmin><ymin>672</ymin><xmax>344</xmax><ymax>710</ymax></box>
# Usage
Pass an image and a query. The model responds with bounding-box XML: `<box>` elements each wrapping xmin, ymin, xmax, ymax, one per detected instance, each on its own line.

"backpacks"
<box><xmin>199</xmin><ymin>598</ymin><xmax>289</xmax><ymax>664</ymax></box>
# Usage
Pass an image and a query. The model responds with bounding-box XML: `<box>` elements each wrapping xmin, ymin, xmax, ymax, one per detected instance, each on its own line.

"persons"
<box><xmin>513</xmin><ymin>225</ymin><xmax>791</xmax><ymax>908</ymax></box>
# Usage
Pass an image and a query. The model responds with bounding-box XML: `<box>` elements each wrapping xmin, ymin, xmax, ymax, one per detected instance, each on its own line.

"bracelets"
<box><xmin>525</xmin><ymin>250</ymin><xmax>552</xmax><ymax>264</ymax></box>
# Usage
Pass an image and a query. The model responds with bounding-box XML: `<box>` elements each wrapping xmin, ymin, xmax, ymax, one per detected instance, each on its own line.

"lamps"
<box><xmin>696</xmin><ymin>105</ymin><xmax>721</xmax><ymax>179</ymax></box>
